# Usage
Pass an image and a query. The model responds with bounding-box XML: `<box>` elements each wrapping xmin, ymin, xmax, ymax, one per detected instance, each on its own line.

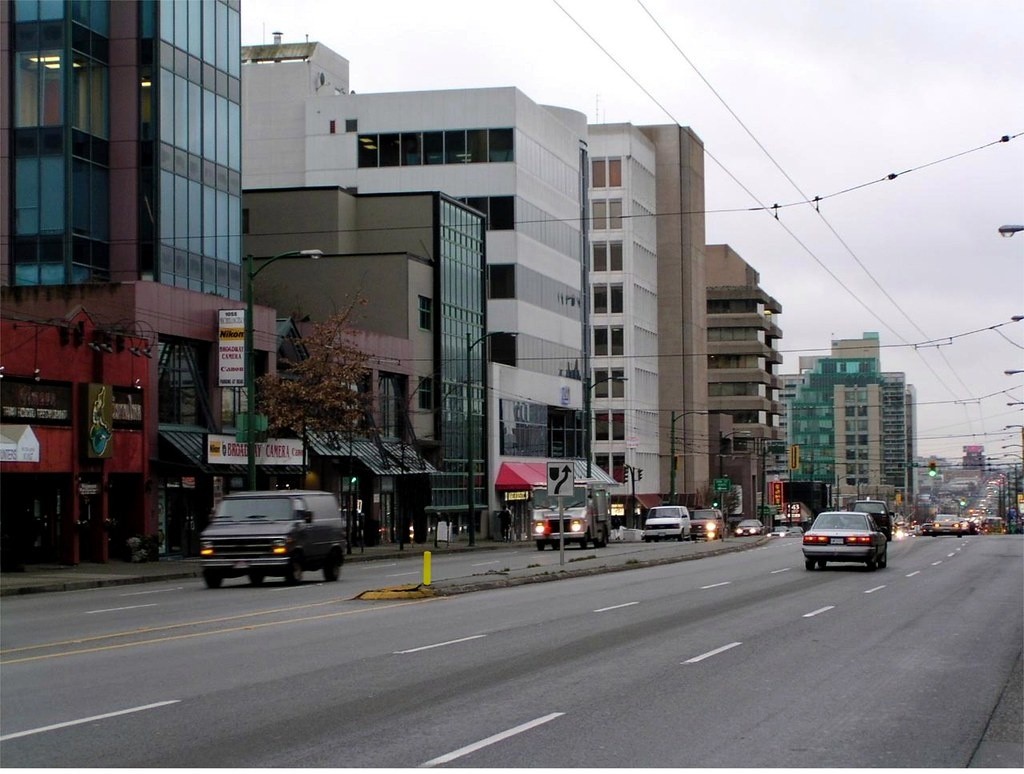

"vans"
<box><xmin>689</xmin><ymin>510</ymin><xmax>724</xmax><ymax>540</ymax></box>
<box><xmin>644</xmin><ymin>506</ymin><xmax>691</xmax><ymax>543</ymax></box>
<box><xmin>850</xmin><ymin>500</ymin><xmax>895</xmax><ymax>542</ymax></box>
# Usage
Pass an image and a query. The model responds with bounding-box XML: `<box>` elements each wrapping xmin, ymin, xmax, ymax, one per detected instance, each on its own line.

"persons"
<box><xmin>497</xmin><ymin>505</ymin><xmax>511</xmax><ymax>543</ymax></box>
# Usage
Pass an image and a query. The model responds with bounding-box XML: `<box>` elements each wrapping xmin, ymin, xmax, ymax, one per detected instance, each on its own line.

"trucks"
<box><xmin>532</xmin><ymin>481</ymin><xmax>610</xmax><ymax>551</ymax></box>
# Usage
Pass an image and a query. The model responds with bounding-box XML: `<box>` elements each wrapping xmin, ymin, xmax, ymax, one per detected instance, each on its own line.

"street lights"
<box><xmin>719</xmin><ymin>430</ymin><xmax>751</xmax><ymax>509</ymax></box>
<box><xmin>670</xmin><ymin>410</ymin><xmax>709</xmax><ymax>504</ymax></box>
<box><xmin>467</xmin><ymin>331</ymin><xmax>519</xmax><ymax>547</ymax></box>
<box><xmin>245</xmin><ymin>249</ymin><xmax>323</xmax><ymax>490</ymax></box>
<box><xmin>585</xmin><ymin>377</ymin><xmax>630</xmax><ymax>479</ymax></box>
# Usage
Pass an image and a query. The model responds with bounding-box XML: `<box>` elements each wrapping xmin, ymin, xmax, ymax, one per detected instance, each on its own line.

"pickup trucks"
<box><xmin>200</xmin><ymin>489</ymin><xmax>346</xmax><ymax>587</ymax></box>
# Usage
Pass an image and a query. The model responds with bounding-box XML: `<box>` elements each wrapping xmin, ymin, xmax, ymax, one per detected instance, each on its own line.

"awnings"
<box><xmin>495</xmin><ymin>462</ymin><xmax>548</xmax><ymax>492</ymax></box>
<box><xmin>296</xmin><ymin>428</ymin><xmax>362</xmax><ymax>458</ymax></box>
<box><xmin>636</xmin><ymin>494</ymin><xmax>663</xmax><ymax>508</ymax></box>
<box><xmin>571</xmin><ymin>460</ymin><xmax>620</xmax><ymax>487</ymax></box>
<box><xmin>158</xmin><ymin>430</ymin><xmax>310</xmax><ymax>476</ymax></box>
<box><xmin>344</xmin><ymin>440</ymin><xmax>437</xmax><ymax>474</ymax></box>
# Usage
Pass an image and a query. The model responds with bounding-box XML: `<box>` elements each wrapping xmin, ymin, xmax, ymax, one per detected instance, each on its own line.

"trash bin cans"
<box><xmin>437</xmin><ymin>513</ymin><xmax>453</xmax><ymax>543</ymax></box>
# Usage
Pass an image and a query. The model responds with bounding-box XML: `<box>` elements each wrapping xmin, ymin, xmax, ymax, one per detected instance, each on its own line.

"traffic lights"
<box><xmin>712</xmin><ymin>497</ymin><xmax>718</xmax><ymax>507</ymax></box>
<box><xmin>622</xmin><ymin>465</ymin><xmax>628</xmax><ymax>484</ymax></box>
<box><xmin>638</xmin><ymin>468</ymin><xmax>642</xmax><ymax>481</ymax></box>
<box><xmin>930</xmin><ymin>461</ymin><xmax>937</xmax><ymax>477</ymax></box>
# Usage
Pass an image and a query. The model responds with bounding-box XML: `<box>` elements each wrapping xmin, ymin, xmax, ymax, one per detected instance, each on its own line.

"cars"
<box><xmin>766</xmin><ymin>526</ymin><xmax>804</xmax><ymax>539</ymax></box>
<box><xmin>918</xmin><ymin>506</ymin><xmax>1007</xmax><ymax>536</ymax></box>
<box><xmin>735</xmin><ymin>519</ymin><xmax>765</xmax><ymax>536</ymax></box>
<box><xmin>802</xmin><ymin>511</ymin><xmax>887</xmax><ymax>571</ymax></box>
<box><xmin>933</xmin><ymin>514</ymin><xmax>962</xmax><ymax>537</ymax></box>
